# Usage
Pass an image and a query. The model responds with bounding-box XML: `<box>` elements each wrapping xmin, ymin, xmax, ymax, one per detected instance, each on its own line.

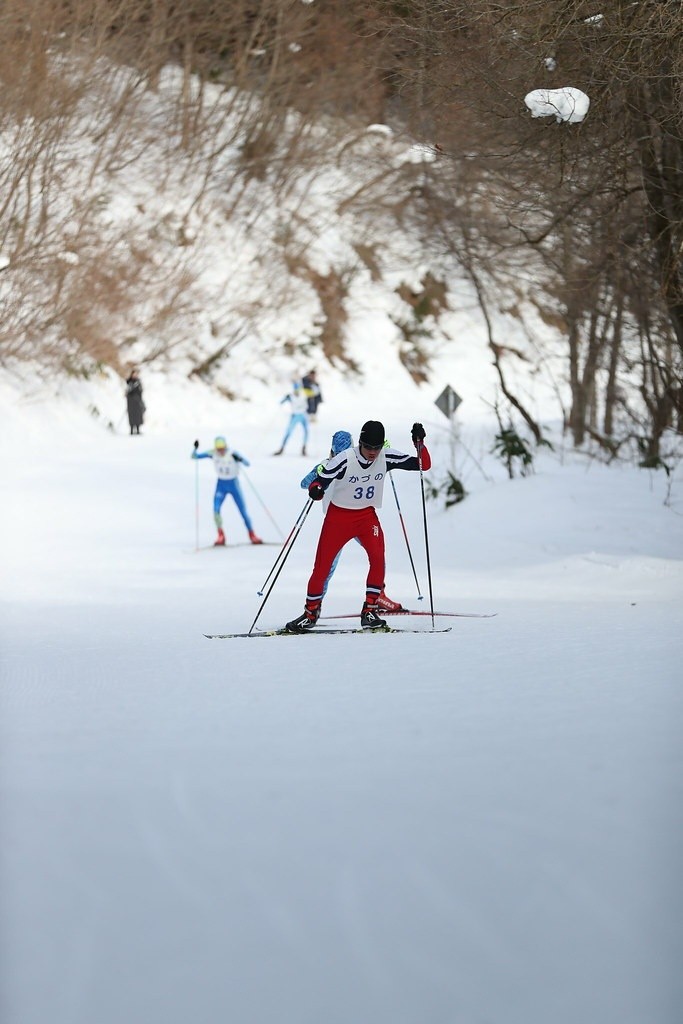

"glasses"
<box><xmin>362</xmin><ymin>445</ymin><xmax>381</xmax><ymax>450</ymax></box>
<box><xmin>333</xmin><ymin>452</ymin><xmax>339</xmax><ymax>456</ymax></box>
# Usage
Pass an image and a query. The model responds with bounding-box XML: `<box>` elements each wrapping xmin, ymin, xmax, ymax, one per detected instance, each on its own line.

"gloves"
<box><xmin>308</xmin><ymin>482</ymin><xmax>325</xmax><ymax>500</ymax></box>
<box><xmin>410</xmin><ymin>423</ymin><xmax>426</xmax><ymax>445</ymax></box>
<box><xmin>231</xmin><ymin>452</ymin><xmax>241</xmax><ymax>461</ymax></box>
<box><xmin>194</xmin><ymin>441</ymin><xmax>198</xmax><ymax>449</ymax></box>
<box><xmin>317</xmin><ymin>463</ymin><xmax>325</xmax><ymax>473</ymax></box>
<box><xmin>383</xmin><ymin>440</ymin><xmax>391</xmax><ymax>447</ymax></box>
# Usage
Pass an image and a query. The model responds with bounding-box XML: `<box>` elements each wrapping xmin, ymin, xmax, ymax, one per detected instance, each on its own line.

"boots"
<box><xmin>249</xmin><ymin>530</ymin><xmax>262</xmax><ymax>543</ymax></box>
<box><xmin>214</xmin><ymin>529</ymin><xmax>224</xmax><ymax>546</ymax></box>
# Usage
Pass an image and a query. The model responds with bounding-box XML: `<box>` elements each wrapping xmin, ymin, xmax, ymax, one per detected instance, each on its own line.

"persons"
<box><xmin>190</xmin><ymin>435</ymin><xmax>263</xmax><ymax>546</ymax></box>
<box><xmin>121</xmin><ymin>368</ymin><xmax>148</xmax><ymax>436</ymax></box>
<box><xmin>302</xmin><ymin>370</ymin><xmax>325</xmax><ymax>424</ymax></box>
<box><xmin>284</xmin><ymin>419</ymin><xmax>432</xmax><ymax>632</ymax></box>
<box><xmin>271</xmin><ymin>379</ymin><xmax>320</xmax><ymax>456</ymax></box>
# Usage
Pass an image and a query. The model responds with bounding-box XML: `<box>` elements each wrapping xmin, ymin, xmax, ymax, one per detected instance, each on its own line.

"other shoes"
<box><xmin>302</xmin><ymin>446</ymin><xmax>307</xmax><ymax>456</ymax></box>
<box><xmin>274</xmin><ymin>445</ymin><xmax>284</xmax><ymax>455</ymax></box>
<box><xmin>377</xmin><ymin>587</ymin><xmax>402</xmax><ymax>611</ymax></box>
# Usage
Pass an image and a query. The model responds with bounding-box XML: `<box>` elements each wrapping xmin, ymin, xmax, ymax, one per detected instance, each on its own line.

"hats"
<box><xmin>215</xmin><ymin>436</ymin><xmax>226</xmax><ymax>449</ymax></box>
<box><xmin>331</xmin><ymin>431</ymin><xmax>353</xmax><ymax>452</ymax></box>
<box><xmin>360</xmin><ymin>420</ymin><xmax>385</xmax><ymax>447</ymax></box>
<box><xmin>294</xmin><ymin>383</ymin><xmax>300</xmax><ymax>391</ymax></box>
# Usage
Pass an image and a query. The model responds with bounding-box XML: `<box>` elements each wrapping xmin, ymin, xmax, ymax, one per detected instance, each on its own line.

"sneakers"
<box><xmin>360</xmin><ymin>601</ymin><xmax>386</xmax><ymax>628</ymax></box>
<box><xmin>287</xmin><ymin>605</ymin><xmax>321</xmax><ymax>630</ymax></box>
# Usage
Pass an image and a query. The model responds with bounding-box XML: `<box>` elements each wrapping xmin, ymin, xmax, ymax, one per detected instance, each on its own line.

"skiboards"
<box><xmin>203</xmin><ymin>627</ymin><xmax>456</xmax><ymax>640</ymax></box>
<box><xmin>193</xmin><ymin>540</ymin><xmax>291</xmax><ymax>548</ymax></box>
<box><xmin>323</xmin><ymin>608</ymin><xmax>503</xmax><ymax>620</ymax></box>
